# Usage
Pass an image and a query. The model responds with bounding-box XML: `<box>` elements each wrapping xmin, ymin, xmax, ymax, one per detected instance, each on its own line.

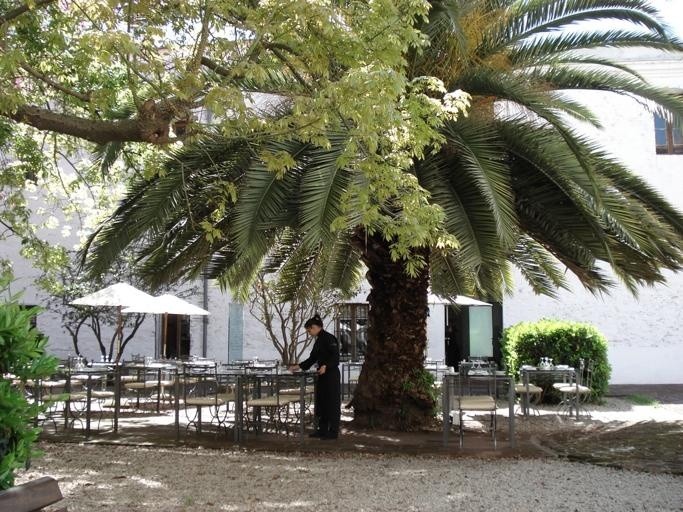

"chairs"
<box><xmin>19</xmin><ymin>353</ymin><xmax>365</xmax><ymax>452</ymax></box>
<box><xmin>420</xmin><ymin>354</ymin><xmax>597</xmax><ymax>450</ymax></box>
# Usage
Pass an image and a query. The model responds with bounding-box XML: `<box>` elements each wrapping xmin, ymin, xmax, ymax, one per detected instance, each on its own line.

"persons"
<box><xmin>290</xmin><ymin>315</ymin><xmax>342</xmax><ymax>439</ymax></box>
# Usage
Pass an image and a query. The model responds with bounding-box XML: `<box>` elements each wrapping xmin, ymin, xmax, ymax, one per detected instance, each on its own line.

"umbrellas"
<box><xmin>121</xmin><ymin>293</ymin><xmax>210</xmax><ymax>360</ymax></box>
<box><xmin>68</xmin><ymin>281</ymin><xmax>155</xmax><ymax>359</ymax></box>
<box><xmin>429</xmin><ymin>289</ymin><xmax>493</xmax><ymax>366</ymax></box>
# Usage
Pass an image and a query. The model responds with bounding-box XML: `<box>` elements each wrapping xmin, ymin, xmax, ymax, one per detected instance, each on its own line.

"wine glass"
<box><xmin>145</xmin><ymin>357</ymin><xmax>152</xmax><ymax>366</ymax></box>
<box><xmin>470</xmin><ymin>359</ymin><xmax>483</xmax><ymax>374</ymax></box>
<box><xmin>74</xmin><ymin>357</ymin><xmax>84</xmax><ymax>371</ymax></box>
<box><xmin>538</xmin><ymin>357</ymin><xmax>553</xmax><ymax>370</ymax></box>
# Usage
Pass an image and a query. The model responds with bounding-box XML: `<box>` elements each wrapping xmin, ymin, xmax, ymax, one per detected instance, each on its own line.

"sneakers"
<box><xmin>310</xmin><ymin>430</ymin><xmax>340</xmax><ymax>440</ymax></box>
